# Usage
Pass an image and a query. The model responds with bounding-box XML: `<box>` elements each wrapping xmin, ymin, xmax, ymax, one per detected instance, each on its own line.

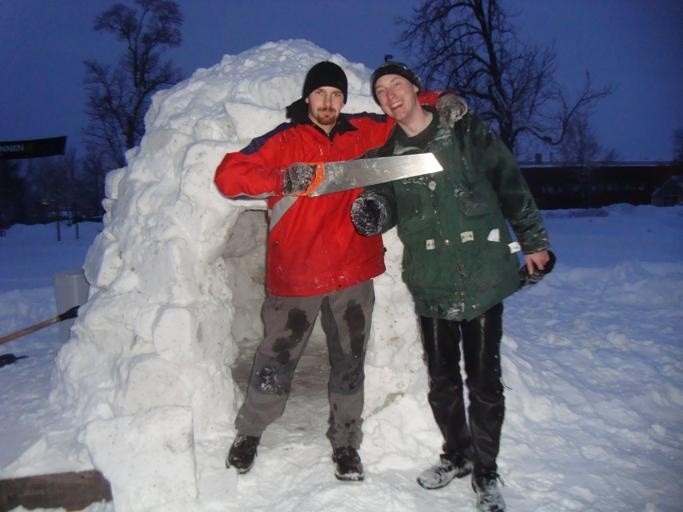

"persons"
<box><xmin>212</xmin><ymin>59</ymin><xmax>471</xmax><ymax>483</ymax></box>
<box><xmin>349</xmin><ymin>62</ymin><xmax>556</xmax><ymax>511</ymax></box>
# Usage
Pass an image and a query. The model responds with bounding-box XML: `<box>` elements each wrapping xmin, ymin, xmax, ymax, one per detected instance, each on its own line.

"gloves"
<box><xmin>437</xmin><ymin>95</ymin><xmax>467</xmax><ymax>129</ymax></box>
<box><xmin>519</xmin><ymin>250</ymin><xmax>555</xmax><ymax>288</ymax></box>
<box><xmin>284</xmin><ymin>163</ymin><xmax>314</xmax><ymax>194</ymax></box>
<box><xmin>351</xmin><ymin>197</ymin><xmax>384</xmax><ymax>236</ymax></box>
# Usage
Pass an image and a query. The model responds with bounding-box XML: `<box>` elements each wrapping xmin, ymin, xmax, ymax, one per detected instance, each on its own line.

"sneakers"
<box><xmin>418</xmin><ymin>454</ymin><xmax>472</xmax><ymax>489</ymax></box>
<box><xmin>226</xmin><ymin>434</ymin><xmax>261</xmax><ymax>474</ymax></box>
<box><xmin>472</xmin><ymin>471</ymin><xmax>506</xmax><ymax>512</ymax></box>
<box><xmin>332</xmin><ymin>446</ymin><xmax>365</xmax><ymax>481</ymax></box>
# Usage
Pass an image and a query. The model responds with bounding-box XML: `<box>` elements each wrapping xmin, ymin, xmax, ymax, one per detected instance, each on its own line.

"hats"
<box><xmin>303</xmin><ymin>61</ymin><xmax>347</xmax><ymax>104</ymax></box>
<box><xmin>371</xmin><ymin>62</ymin><xmax>421</xmax><ymax>105</ymax></box>
